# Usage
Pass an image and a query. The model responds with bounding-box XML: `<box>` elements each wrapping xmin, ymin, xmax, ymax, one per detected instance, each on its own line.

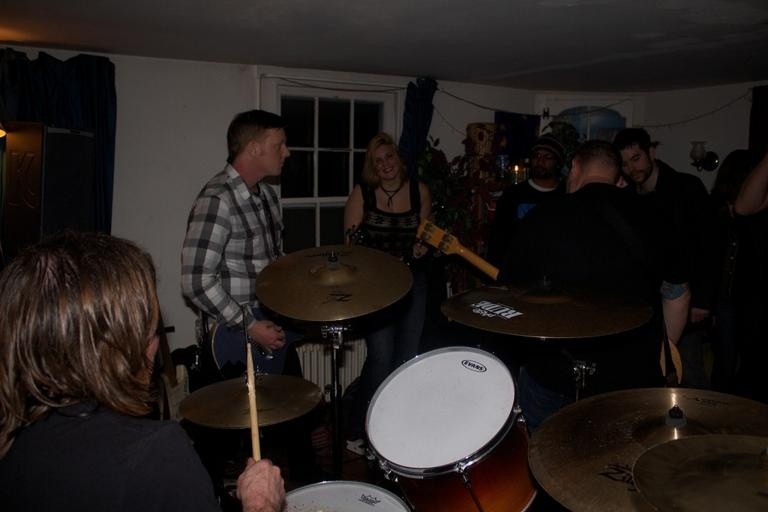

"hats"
<box><xmin>529</xmin><ymin>134</ymin><xmax>563</xmax><ymax>158</ymax></box>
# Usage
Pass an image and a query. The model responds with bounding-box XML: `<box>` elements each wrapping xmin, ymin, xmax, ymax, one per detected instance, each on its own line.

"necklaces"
<box><xmin>375</xmin><ymin>181</ymin><xmax>408</xmax><ymax>210</ymax></box>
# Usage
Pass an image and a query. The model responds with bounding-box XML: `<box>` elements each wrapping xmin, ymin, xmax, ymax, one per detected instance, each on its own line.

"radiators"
<box><xmin>294</xmin><ymin>338</ymin><xmax>368</xmax><ymax>402</ymax></box>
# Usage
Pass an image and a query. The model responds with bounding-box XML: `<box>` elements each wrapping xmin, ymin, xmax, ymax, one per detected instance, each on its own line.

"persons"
<box><xmin>0</xmin><ymin>234</ymin><xmax>288</xmax><ymax>512</ymax></box>
<box><xmin>610</xmin><ymin>127</ymin><xmax>725</xmax><ymax>389</ymax></box>
<box><xmin>711</xmin><ymin>150</ymin><xmax>767</xmax><ymax>404</ymax></box>
<box><xmin>181</xmin><ymin>109</ymin><xmax>293</xmax><ymax>375</ymax></box>
<box><xmin>343</xmin><ymin>133</ymin><xmax>432</xmax><ymax>455</ymax></box>
<box><xmin>516</xmin><ymin>140</ymin><xmax>693</xmax><ymax>436</ymax></box>
<box><xmin>490</xmin><ymin>134</ymin><xmax>568</xmax><ymax>283</ymax></box>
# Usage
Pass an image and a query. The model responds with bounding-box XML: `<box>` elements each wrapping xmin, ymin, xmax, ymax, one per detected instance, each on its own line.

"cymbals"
<box><xmin>179</xmin><ymin>372</ymin><xmax>323</xmax><ymax>429</ymax></box>
<box><xmin>527</xmin><ymin>387</ymin><xmax>767</xmax><ymax>511</ymax></box>
<box><xmin>634</xmin><ymin>433</ymin><xmax>768</xmax><ymax>512</ymax></box>
<box><xmin>254</xmin><ymin>244</ymin><xmax>414</xmax><ymax>321</ymax></box>
<box><xmin>441</xmin><ymin>275</ymin><xmax>655</xmax><ymax>340</ymax></box>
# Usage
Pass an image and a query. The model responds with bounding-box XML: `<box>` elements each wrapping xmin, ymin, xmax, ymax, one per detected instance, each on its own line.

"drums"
<box><xmin>364</xmin><ymin>345</ymin><xmax>539</xmax><ymax>512</ymax></box>
<box><xmin>280</xmin><ymin>481</ymin><xmax>410</xmax><ymax>512</ymax></box>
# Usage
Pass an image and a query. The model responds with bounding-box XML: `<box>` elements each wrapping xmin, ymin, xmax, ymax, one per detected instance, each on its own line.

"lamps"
<box><xmin>688</xmin><ymin>140</ymin><xmax>718</xmax><ymax>171</ymax></box>
